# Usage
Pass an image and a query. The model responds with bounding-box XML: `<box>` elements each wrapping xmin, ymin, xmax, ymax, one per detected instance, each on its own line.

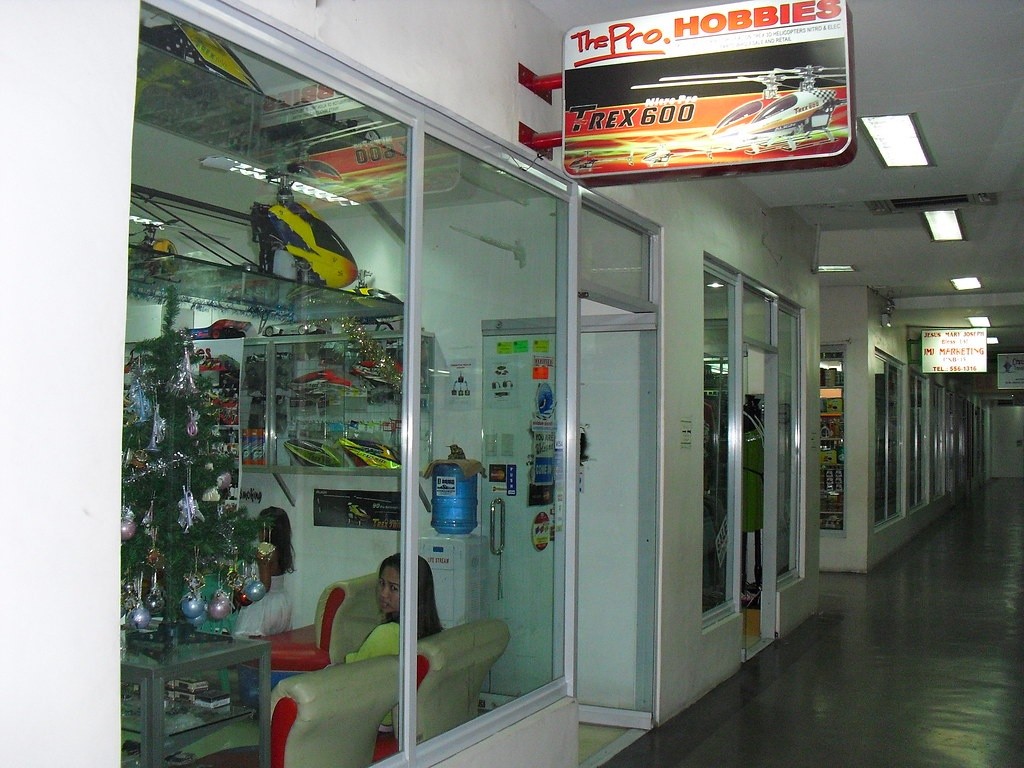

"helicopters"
<box><xmin>337</xmin><ymin>267</ymin><xmax>404</xmax><ymax>332</ymax></box>
<box><xmin>658</xmin><ymin>65</ymin><xmax>849</xmax><ymax>154</ymax></box>
<box><xmin>629</xmin><ymin>73</ymin><xmax>847</xmax><ymax>151</ymax></box>
<box><xmin>250</xmin><ymin>163</ymin><xmax>359</xmax><ymax>291</ymax></box>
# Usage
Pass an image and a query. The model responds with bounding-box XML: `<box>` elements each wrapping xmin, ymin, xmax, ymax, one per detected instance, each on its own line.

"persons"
<box><xmin>234</xmin><ymin>507</ymin><xmax>295</xmax><ymax>641</ymax></box>
<box><xmin>343</xmin><ymin>553</ymin><xmax>441</xmax><ymax>734</ymax></box>
<box><xmin>345</xmin><ymin>502</ymin><xmax>364</xmax><ymax>526</ymax></box>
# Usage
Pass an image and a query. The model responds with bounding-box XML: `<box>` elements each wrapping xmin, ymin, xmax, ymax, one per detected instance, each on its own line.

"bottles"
<box><xmin>431</xmin><ymin>462</ymin><xmax>479</xmax><ymax>534</ymax></box>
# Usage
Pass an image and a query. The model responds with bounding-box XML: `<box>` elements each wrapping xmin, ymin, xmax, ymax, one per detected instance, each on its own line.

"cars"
<box><xmin>262</xmin><ymin>318</ymin><xmax>331</xmax><ymax>336</ymax></box>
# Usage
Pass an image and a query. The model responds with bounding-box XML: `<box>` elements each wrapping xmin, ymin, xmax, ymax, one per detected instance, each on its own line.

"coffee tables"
<box><xmin>120</xmin><ymin>619</ymin><xmax>272</xmax><ymax>768</ymax></box>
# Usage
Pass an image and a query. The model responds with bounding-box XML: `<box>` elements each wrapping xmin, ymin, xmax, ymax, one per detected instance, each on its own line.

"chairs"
<box><xmin>178</xmin><ymin>654</ymin><xmax>427</xmax><ymax>768</ymax></box>
<box><xmin>358</xmin><ymin>616</ymin><xmax>508</xmax><ymax>764</ymax></box>
<box><xmin>239</xmin><ymin>567</ymin><xmax>387</xmax><ymax>670</ymax></box>
